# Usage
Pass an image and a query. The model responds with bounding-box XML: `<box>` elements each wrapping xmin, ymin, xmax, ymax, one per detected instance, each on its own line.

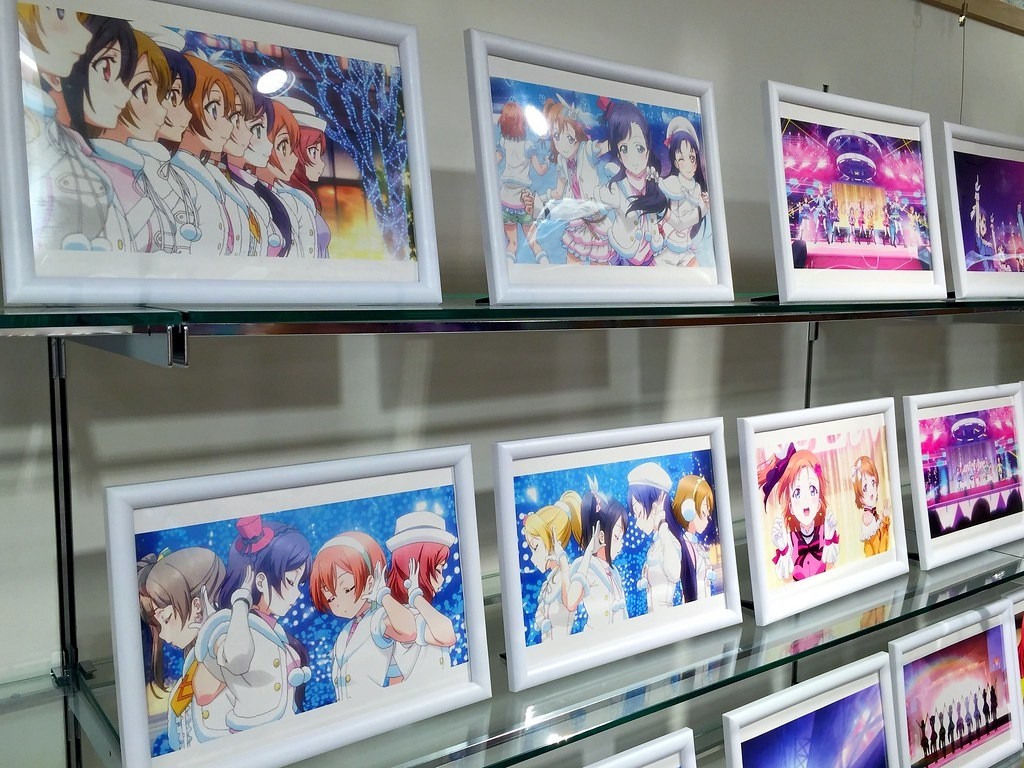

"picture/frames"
<box><xmin>493</xmin><ymin>415</ymin><xmax>744</xmax><ymax>691</ymax></box>
<box><xmin>105</xmin><ymin>445</ymin><xmax>496</xmax><ymax>768</ymax></box>
<box><xmin>1002</xmin><ymin>586</ymin><xmax>1024</xmax><ymax>744</ymax></box>
<box><xmin>761</xmin><ymin>80</ymin><xmax>949</xmax><ymax>305</ymax></box>
<box><xmin>723</xmin><ymin>651</ymin><xmax>900</xmax><ymax>768</ymax></box>
<box><xmin>2</xmin><ymin>0</ymin><xmax>445</xmax><ymax>303</ymax></box>
<box><xmin>887</xmin><ymin>600</ymin><xmax>1024</xmax><ymax>768</ymax></box>
<box><xmin>934</xmin><ymin>119</ymin><xmax>1024</xmax><ymax>304</ymax></box>
<box><xmin>463</xmin><ymin>29</ymin><xmax>737</xmax><ymax>301</ymax></box>
<box><xmin>736</xmin><ymin>397</ymin><xmax>910</xmax><ymax>628</ymax></box>
<box><xmin>902</xmin><ymin>380</ymin><xmax>1024</xmax><ymax>569</ymax></box>
<box><xmin>582</xmin><ymin>726</ymin><xmax>697</xmax><ymax>768</ymax></box>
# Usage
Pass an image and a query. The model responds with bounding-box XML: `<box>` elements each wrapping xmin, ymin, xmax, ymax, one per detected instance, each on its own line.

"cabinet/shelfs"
<box><xmin>0</xmin><ymin>0</ymin><xmax>1024</xmax><ymax>768</ymax></box>
